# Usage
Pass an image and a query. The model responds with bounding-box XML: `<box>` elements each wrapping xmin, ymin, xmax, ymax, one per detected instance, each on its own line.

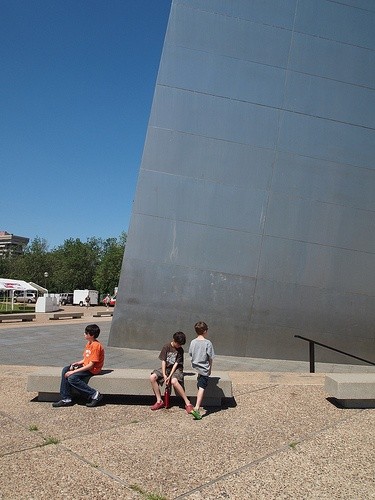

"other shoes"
<box><xmin>52</xmin><ymin>400</ymin><xmax>73</xmax><ymax>407</ymax></box>
<box><xmin>86</xmin><ymin>395</ymin><xmax>103</xmax><ymax>407</ymax></box>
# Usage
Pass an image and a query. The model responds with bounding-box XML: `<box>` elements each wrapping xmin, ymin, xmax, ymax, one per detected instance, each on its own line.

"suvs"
<box><xmin>14</xmin><ymin>293</ymin><xmax>39</xmax><ymax>303</ymax></box>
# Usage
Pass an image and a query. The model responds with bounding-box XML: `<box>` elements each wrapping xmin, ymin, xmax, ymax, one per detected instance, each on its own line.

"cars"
<box><xmin>100</xmin><ymin>294</ymin><xmax>107</xmax><ymax>305</ymax></box>
<box><xmin>103</xmin><ymin>294</ymin><xmax>117</xmax><ymax>307</ymax></box>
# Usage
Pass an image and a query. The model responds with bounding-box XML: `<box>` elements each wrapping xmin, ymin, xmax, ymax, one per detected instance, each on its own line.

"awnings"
<box><xmin>0</xmin><ymin>277</ymin><xmax>48</xmax><ymax>312</ymax></box>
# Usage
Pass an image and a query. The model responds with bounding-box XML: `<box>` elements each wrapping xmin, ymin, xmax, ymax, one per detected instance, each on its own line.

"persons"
<box><xmin>84</xmin><ymin>295</ymin><xmax>91</xmax><ymax>307</ymax></box>
<box><xmin>104</xmin><ymin>293</ymin><xmax>112</xmax><ymax>309</ymax></box>
<box><xmin>149</xmin><ymin>331</ymin><xmax>195</xmax><ymax>415</ymax></box>
<box><xmin>51</xmin><ymin>324</ymin><xmax>105</xmax><ymax>408</ymax></box>
<box><xmin>190</xmin><ymin>321</ymin><xmax>216</xmax><ymax>420</ymax></box>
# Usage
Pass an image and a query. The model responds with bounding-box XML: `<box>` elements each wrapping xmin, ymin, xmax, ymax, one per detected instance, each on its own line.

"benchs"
<box><xmin>93</xmin><ymin>311</ymin><xmax>113</xmax><ymax>317</ymax></box>
<box><xmin>48</xmin><ymin>313</ymin><xmax>83</xmax><ymax>320</ymax></box>
<box><xmin>324</xmin><ymin>374</ymin><xmax>375</xmax><ymax>408</ymax></box>
<box><xmin>0</xmin><ymin>314</ymin><xmax>36</xmax><ymax>323</ymax></box>
<box><xmin>26</xmin><ymin>370</ymin><xmax>229</xmax><ymax>409</ymax></box>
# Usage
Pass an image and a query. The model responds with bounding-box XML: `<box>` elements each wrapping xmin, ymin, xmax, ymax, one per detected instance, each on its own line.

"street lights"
<box><xmin>44</xmin><ymin>272</ymin><xmax>48</xmax><ymax>290</ymax></box>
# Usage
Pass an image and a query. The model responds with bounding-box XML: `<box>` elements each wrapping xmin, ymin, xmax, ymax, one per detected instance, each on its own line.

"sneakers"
<box><xmin>185</xmin><ymin>404</ymin><xmax>194</xmax><ymax>414</ymax></box>
<box><xmin>151</xmin><ymin>400</ymin><xmax>163</xmax><ymax>410</ymax></box>
<box><xmin>190</xmin><ymin>409</ymin><xmax>203</xmax><ymax>419</ymax></box>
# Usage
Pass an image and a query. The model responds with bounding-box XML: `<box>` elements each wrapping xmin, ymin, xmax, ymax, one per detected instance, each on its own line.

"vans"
<box><xmin>58</xmin><ymin>292</ymin><xmax>74</xmax><ymax>305</ymax></box>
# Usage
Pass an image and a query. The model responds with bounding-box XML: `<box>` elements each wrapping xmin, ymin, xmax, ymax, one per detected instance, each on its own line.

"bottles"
<box><xmin>164</xmin><ymin>387</ymin><xmax>170</xmax><ymax>409</ymax></box>
